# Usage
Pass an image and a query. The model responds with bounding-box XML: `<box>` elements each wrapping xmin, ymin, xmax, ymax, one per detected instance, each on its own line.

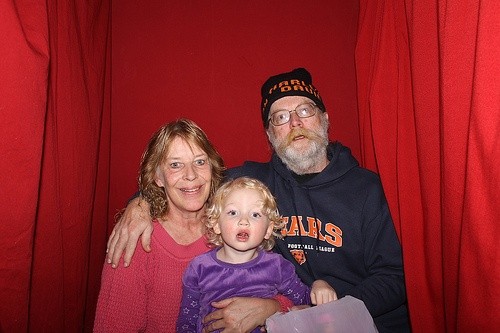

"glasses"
<box><xmin>267</xmin><ymin>102</ymin><xmax>317</xmax><ymax>126</ymax></box>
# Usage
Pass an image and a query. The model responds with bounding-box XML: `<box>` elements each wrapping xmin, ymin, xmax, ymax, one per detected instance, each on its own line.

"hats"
<box><xmin>260</xmin><ymin>68</ymin><xmax>326</xmax><ymax>129</ymax></box>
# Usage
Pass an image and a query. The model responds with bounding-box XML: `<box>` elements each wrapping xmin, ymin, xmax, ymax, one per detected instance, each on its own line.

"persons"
<box><xmin>106</xmin><ymin>66</ymin><xmax>412</xmax><ymax>333</ymax></box>
<box><xmin>174</xmin><ymin>174</ymin><xmax>337</xmax><ymax>333</ymax></box>
<box><xmin>92</xmin><ymin>116</ymin><xmax>296</xmax><ymax>333</ymax></box>
